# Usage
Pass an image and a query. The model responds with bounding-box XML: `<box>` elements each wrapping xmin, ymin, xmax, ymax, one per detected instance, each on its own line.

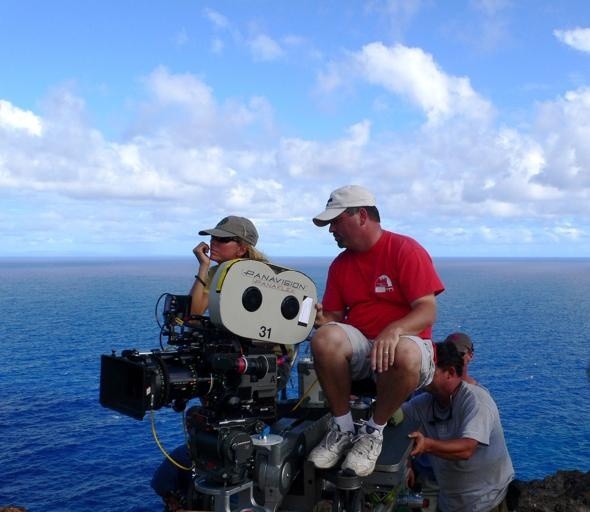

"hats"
<box><xmin>445</xmin><ymin>333</ymin><xmax>472</xmax><ymax>359</ymax></box>
<box><xmin>313</xmin><ymin>186</ymin><xmax>377</xmax><ymax>226</ymax></box>
<box><xmin>199</xmin><ymin>216</ymin><xmax>258</xmax><ymax>247</ymax></box>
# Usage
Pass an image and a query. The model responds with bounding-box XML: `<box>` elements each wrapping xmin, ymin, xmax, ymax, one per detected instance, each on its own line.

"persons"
<box><xmin>388</xmin><ymin>339</ymin><xmax>516</xmax><ymax>511</ymax></box>
<box><xmin>186</xmin><ymin>215</ymin><xmax>297</xmax><ymax>372</ymax></box>
<box><xmin>442</xmin><ymin>332</ymin><xmax>491</xmax><ymax>395</ymax></box>
<box><xmin>304</xmin><ymin>184</ymin><xmax>446</xmax><ymax>480</ymax></box>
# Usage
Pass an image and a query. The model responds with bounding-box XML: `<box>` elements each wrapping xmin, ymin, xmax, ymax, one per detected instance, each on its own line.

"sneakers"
<box><xmin>307</xmin><ymin>419</ymin><xmax>383</xmax><ymax>477</ymax></box>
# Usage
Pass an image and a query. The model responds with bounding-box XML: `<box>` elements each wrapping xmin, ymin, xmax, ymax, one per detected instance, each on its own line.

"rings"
<box><xmin>383</xmin><ymin>351</ymin><xmax>388</xmax><ymax>356</ymax></box>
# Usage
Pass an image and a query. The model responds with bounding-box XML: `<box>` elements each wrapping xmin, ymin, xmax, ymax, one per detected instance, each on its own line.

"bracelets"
<box><xmin>195</xmin><ymin>275</ymin><xmax>206</xmax><ymax>287</ymax></box>
